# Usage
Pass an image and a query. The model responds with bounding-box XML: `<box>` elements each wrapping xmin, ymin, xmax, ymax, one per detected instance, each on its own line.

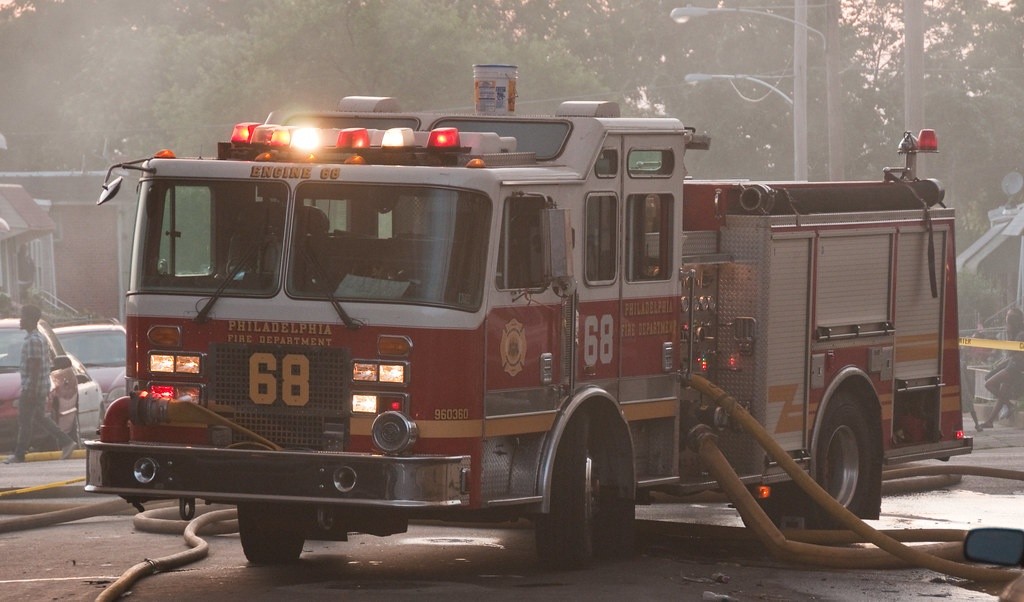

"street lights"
<box><xmin>685</xmin><ymin>0</ymin><xmax>814</xmax><ymax>180</ymax></box>
<box><xmin>671</xmin><ymin>0</ymin><xmax>846</xmax><ymax>182</ymax></box>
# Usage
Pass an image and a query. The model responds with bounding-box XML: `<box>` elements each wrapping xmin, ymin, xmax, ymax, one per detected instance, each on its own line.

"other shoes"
<box><xmin>999</xmin><ymin>402</ymin><xmax>1016</xmax><ymax>420</ymax></box>
<box><xmin>980</xmin><ymin>421</ymin><xmax>993</xmax><ymax>428</ymax></box>
<box><xmin>974</xmin><ymin>424</ymin><xmax>982</xmax><ymax>432</ymax></box>
<box><xmin>60</xmin><ymin>441</ymin><xmax>78</xmax><ymax>460</ymax></box>
<box><xmin>3</xmin><ymin>455</ymin><xmax>25</xmax><ymax>464</ymax></box>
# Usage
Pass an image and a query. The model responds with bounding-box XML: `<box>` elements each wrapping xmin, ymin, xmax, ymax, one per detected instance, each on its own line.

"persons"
<box><xmin>3</xmin><ymin>305</ymin><xmax>77</xmax><ymax>463</ymax></box>
<box><xmin>979</xmin><ymin>306</ymin><xmax>1024</xmax><ymax>429</ymax></box>
<box><xmin>18</xmin><ymin>244</ymin><xmax>36</xmax><ymax>299</ymax></box>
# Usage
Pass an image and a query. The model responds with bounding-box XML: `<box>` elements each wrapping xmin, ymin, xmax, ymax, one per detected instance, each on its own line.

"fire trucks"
<box><xmin>83</xmin><ymin>96</ymin><xmax>976</xmax><ymax>560</ymax></box>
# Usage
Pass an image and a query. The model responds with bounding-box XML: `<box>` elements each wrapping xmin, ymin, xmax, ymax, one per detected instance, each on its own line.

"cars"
<box><xmin>63</xmin><ymin>350</ymin><xmax>104</xmax><ymax>439</ymax></box>
<box><xmin>50</xmin><ymin>319</ymin><xmax>129</xmax><ymax>434</ymax></box>
<box><xmin>1</xmin><ymin>318</ymin><xmax>78</xmax><ymax>451</ymax></box>
